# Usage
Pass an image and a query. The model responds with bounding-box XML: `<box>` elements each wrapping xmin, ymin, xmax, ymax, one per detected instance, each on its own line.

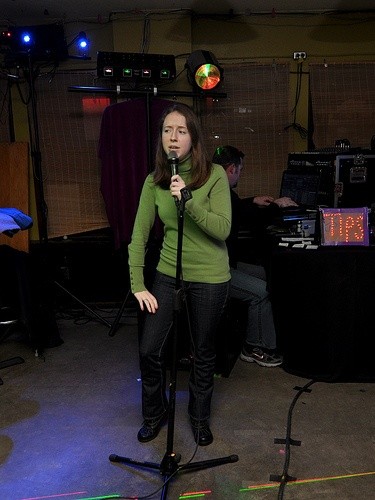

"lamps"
<box><xmin>68</xmin><ymin>31</ymin><xmax>88</xmax><ymax>49</ymax></box>
<box><xmin>186</xmin><ymin>50</ymin><xmax>224</xmax><ymax>92</ymax></box>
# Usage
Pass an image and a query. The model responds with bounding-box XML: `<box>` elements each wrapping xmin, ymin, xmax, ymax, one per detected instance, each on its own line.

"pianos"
<box><xmin>236</xmin><ymin>136</ymin><xmax>375</xmax><ymax>384</ymax></box>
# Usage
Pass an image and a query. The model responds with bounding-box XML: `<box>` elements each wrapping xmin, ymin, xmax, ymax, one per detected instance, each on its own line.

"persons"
<box><xmin>212</xmin><ymin>145</ymin><xmax>298</xmax><ymax>368</ymax></box>
<box><xmin>128</xmin><ymin>104</ymin><xmax>231</xmax><ymax>445</ymax></box>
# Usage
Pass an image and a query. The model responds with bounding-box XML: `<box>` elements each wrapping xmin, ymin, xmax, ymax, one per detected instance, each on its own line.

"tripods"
<box><xmin>108</xmin><ymin>197</ymin><xmax>239</xmax><ymax>500</ymax></box>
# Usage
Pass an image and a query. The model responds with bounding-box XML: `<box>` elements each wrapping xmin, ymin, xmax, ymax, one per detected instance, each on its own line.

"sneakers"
<box><xmin>240</xmin><ymin>349</ymin><xmax>283</xmax><ymax>367</ymax></box>
<box><xmin>137</xmin><ymin>413</ymin><xmax>167</xmax><ymax>442</ymax></box>
<box><xmin>190</xmin><ymin>416</ymin><xmax>213</xmax><ymax>446</ymax></box>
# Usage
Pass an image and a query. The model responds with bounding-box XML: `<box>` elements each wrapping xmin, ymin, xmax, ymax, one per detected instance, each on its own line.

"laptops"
<box><xmin>279</xmin><ymin>170</ymin><xmax>320</xmax><ymax>211</ymax></box>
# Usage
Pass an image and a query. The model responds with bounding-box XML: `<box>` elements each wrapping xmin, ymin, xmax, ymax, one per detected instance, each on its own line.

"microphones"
<box><xmin>167</xmin><ymin>151</ymin><xmax>179</xmax><ymax>204</ymax></box>
